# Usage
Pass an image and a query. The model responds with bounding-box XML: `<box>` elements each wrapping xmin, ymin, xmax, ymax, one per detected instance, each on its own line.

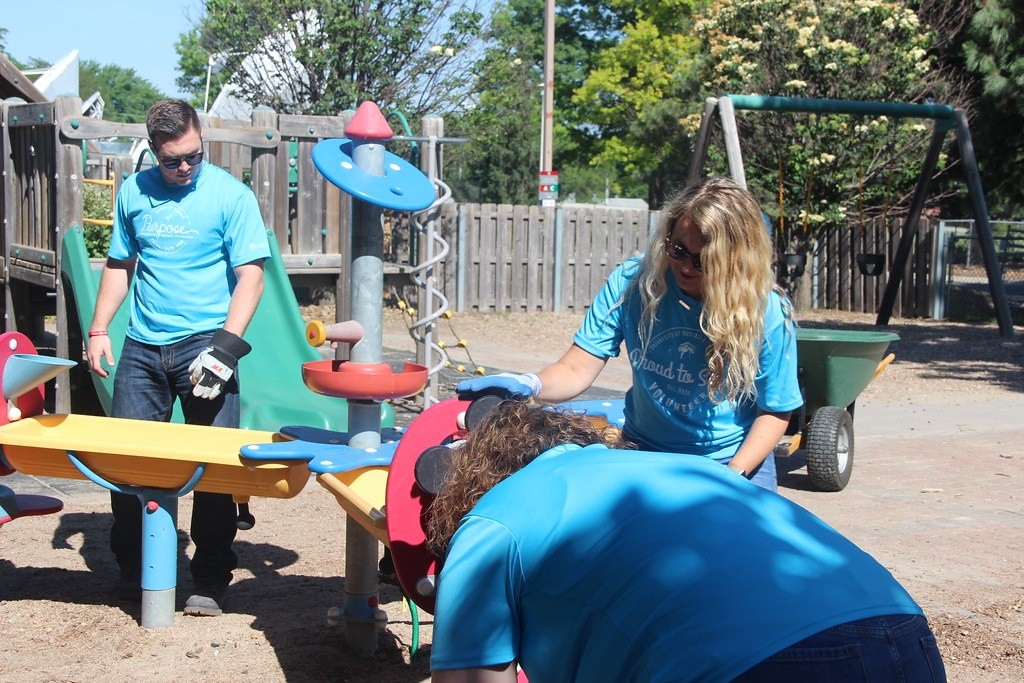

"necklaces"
<box><xmin>669</xmin><ymin>278</ymin><xmax>692</xmax><ymax>311</ymax></box>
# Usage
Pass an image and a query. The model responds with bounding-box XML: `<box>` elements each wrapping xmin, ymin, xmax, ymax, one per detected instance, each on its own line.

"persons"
<box><xmin>453</xmin><ymin>176</ymin><xmax>804</xmax><ymax>494</ymax></box>
<box><xmin>87</xmin><ymin>99</ymin><xmax>272</xmax><ymax>615</ymax></box>
<box><xmin>425</xmin><ymin>396</ymin><xmax>948</xmax><ymax>683</ymax></box>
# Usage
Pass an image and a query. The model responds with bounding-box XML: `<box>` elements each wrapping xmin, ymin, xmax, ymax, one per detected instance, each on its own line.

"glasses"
<box><xmin>664</xmin><ymin>231</ymin><xmax>702</xmax><ymax>270</ymax></box>
<box><xmin>148</xmin><ymin>137</ymin><xmax>203</xmax><ymax>169</ymax></box>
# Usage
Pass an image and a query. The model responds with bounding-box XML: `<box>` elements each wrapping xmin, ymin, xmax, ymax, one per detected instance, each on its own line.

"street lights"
<box><xmin>204</xmin><ymin>46</ymin><xmax>269</xmax><ymax>112</ymax></box>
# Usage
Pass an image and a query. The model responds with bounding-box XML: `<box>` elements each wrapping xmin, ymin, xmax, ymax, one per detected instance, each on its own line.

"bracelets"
<box><xmin>727</xmin><ymin>463</ymin><xmax>747</xmax><ymax>478</ymax></box>
<box><xmin>89</xmin><ymin>331</ymin><xmax>108</xmax><ymax>338</ymax></box>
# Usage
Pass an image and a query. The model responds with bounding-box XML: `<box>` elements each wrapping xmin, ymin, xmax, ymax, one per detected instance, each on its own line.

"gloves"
<box><xmin>188</xmin><ymin>328</ymin><xmax>251</xmax><ymax>400</ymax></box>
<box><xmin>455</xmin><ymin>372</ymin><xmax>542</xmax><ymax>400</ymax></box>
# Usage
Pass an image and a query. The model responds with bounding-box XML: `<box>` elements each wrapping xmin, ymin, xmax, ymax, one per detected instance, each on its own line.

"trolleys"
<box><xmin>773</xmin><ymin>327</ymin><xmax>901</xmax><ymax>493</ymax></box>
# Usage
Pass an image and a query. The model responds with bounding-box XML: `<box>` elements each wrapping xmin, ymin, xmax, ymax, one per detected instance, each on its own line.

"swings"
<box><xmin>772</xmin><ymin>111</ymin><xmax>822</xmax><ymax>278</ymax></box>
<box><xmin>855</xmin><ymin>118</ymin><xmax>900</xmax><ymax>277</ymax></box>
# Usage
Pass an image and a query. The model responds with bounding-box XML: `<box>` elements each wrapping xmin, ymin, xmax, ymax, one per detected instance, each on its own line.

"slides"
<box><xmin>62</xmin><ymin>227</ymin><xmax>395</xmax><ymax>445</ymax></box>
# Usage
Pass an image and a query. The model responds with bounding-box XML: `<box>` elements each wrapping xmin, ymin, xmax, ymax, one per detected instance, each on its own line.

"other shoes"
<box><xmin>185</xmin><ymin>585</ymin><xmax>227</xmax><ymax>615</ymax></box>
<box><xmin>114</xmin><ymin>574</ymin><xmax>142</xmax><ymax>603</ymax></box>
